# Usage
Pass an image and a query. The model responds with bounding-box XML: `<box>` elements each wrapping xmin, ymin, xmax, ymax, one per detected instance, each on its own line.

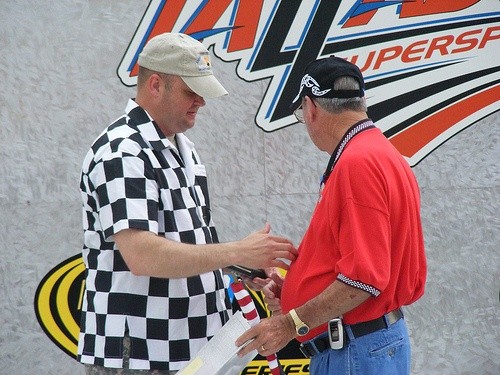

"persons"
<box><xmin>235</xmin><ymin>54</ymin><xmax>427</xmax><ymax>375</ymax></box>
<box><xmin>76</xmin><ymin>32</ymin><xmax>301</xmax><ymax>375</ymax></box>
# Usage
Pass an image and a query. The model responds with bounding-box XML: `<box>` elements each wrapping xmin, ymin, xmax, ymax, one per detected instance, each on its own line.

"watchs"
<box><xmin>288</xmin><ymin>308</ymin><xmax>310</xmax><ymax>336</ymax></box>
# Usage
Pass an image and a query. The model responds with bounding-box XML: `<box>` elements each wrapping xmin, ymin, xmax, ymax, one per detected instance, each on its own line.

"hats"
<box><xmin>137</xmin><ymin>32</ymin><xmax>229</xmax><ymax>99</ymax></box>
<box><xmin>290</xmin><ymin>54</ymin><xmax>365</xmax><ymax>112</ymax></box>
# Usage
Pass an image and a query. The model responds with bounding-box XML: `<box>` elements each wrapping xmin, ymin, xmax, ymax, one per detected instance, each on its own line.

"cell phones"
<box><xmin>328</xmin><ymin>318</ymin><xmax>344</xmax><ymax>349</ymax></box>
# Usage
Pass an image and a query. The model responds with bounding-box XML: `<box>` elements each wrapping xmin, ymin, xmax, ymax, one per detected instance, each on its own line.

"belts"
<box><xmin>300</xmin><ymin>308</ymin><xmax>404</xmax><ymax>359</ymax></box>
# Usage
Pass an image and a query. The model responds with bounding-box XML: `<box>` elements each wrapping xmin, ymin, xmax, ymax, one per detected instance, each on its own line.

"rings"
<box><xmin>261</xmin><ymin>345</ymin><xmax>266</xmax><ymax>351</ymax></box>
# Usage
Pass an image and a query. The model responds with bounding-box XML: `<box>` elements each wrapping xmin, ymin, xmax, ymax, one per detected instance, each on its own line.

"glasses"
<box><xmin>293</xmin><ymin>101</ymin><xmax>306</xmax><ymax>124</ymax></box>
